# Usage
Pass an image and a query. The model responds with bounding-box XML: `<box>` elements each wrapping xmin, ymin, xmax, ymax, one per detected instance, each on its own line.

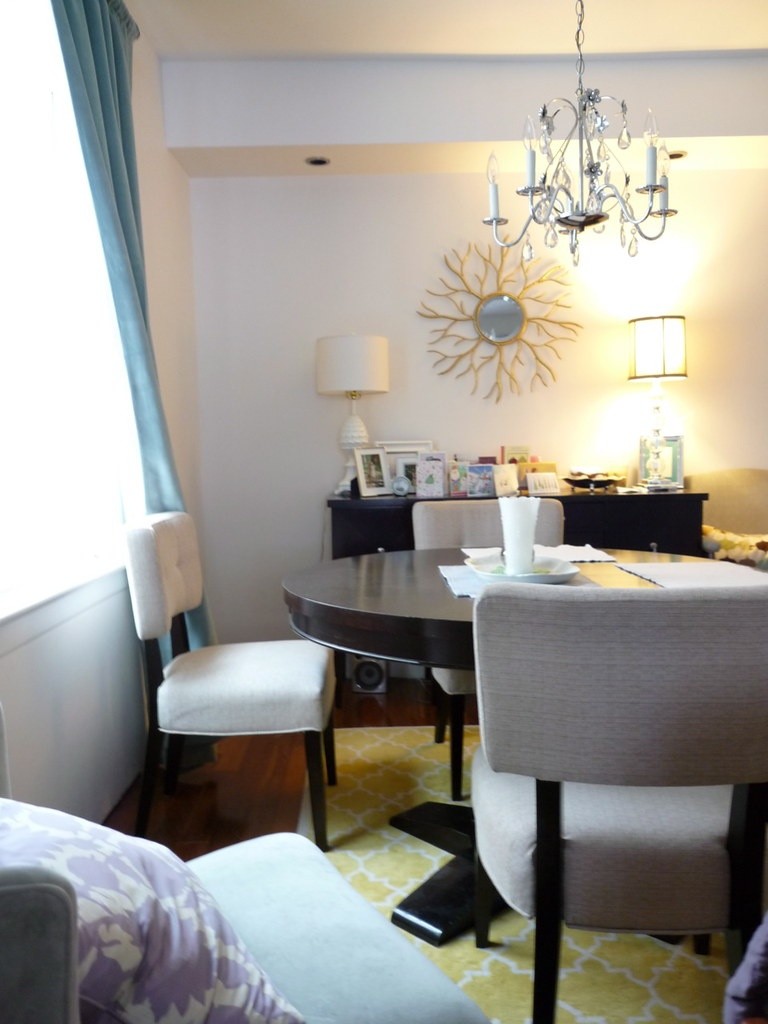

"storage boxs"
<box><xmin>416</xmin><ymin>462</ymin><xmax>448</xmax><ymax>497</ymax></box>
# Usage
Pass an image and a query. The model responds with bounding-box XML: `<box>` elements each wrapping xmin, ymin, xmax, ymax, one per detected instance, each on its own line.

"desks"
<box><xmin>328</xmin><ymin>492</ymin><xmax>710</xmax><ymax>731</ymax></box>
<box><xmin>281</xmin><ymin>548</ymin><xmax>768</xmax><ymax>946</ymax></box>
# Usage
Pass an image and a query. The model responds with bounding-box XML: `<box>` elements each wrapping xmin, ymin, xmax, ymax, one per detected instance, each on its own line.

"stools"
<box><xmin>184</xmin><ymin>833</ymin><xmax>489</xmax><ymax>1024</ymax></box>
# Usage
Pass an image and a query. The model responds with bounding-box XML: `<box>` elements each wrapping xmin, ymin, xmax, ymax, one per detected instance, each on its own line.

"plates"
<box><xmin>562</xmin><ymin>476</ymin><xmax>626</xmax><ymax>488</ymax></box>
<box><xmin>464</xmin><ymin>553</ymin><xmax>581</xmax><ymax>585</ymax></box>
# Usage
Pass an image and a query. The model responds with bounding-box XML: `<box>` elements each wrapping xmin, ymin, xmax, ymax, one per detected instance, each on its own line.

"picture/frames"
<box><xmin>397</xmin><ymin>459</ymin><xmax>416</xmax><ymax>493</ymax></box>
<box><xmin>374</xmin><ymin>440</ymin><xmax>433</xmax><ymax>479</ymax></box>
<box><xmin>638</xmin><ymin>434</ymin><xmax>686</xmax><ymax>488</ymax></box>
<box><xmin>353</xmin><ymin>446</ymin><xmax>392</xmax><ymax>496</ymax></box>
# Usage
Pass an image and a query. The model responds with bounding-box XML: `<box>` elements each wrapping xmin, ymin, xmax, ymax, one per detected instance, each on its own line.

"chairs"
<box><xmin>473</xmin><ymin>569</ymin><xmax>768</xmax><ymax>1024</ymax></box>
<box><xmin>124</xmin><ymin>510</ymin><xmax>339</xmax><ymax>852</ymax></box>
<box><xmin>413</xmin><ymin>499</ymin><xmax>563</xmax><ymax>800</ymax></box>
<box><xmin>0</xmin><ymin>869</ymin><xmax>81</xmax><ymax>1024</ymax></box>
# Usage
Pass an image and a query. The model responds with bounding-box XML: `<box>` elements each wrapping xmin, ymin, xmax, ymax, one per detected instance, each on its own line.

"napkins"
<box><xmin>437</xmin><ymin>563</ymin><xmax>480</xmax><ymax>597</ymax></box>
<box><xmin>461</xmin><ymin>543</ymin><xmax>617</xmax><ymax>565</ymax></box>
<box><xmin>616</xmin><ymin>560</ymin><xmax>768</xmax><ymax>588</ymax></box>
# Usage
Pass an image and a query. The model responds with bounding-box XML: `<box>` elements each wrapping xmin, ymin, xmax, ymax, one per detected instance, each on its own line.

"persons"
<box><xmin>369</xmin><ymin>455</ymin><xmax>384</xmax><ymax>487</ymax></box>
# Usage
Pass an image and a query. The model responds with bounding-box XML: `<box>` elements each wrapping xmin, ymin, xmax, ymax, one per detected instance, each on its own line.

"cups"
<box><xmin>498</xmin><ymin>496</ymin><xmax>542</xmax><ymax>573</ymax></box>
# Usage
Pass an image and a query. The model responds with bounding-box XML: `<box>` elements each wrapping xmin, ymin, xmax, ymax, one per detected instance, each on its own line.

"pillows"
<box><xmin>0</xmin><ymin>796</ymin><xmax>304</xmax><ymax>1024</ymax></box>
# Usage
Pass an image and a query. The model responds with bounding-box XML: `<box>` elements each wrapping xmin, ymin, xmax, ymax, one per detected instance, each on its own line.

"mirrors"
<box><xmin>415</xmin><ymin>239</ymin><xmax>589</xmax><ymax>397</ymax></box>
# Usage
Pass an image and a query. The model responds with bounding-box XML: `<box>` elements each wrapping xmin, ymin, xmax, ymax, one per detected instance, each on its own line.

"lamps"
<box><xmin>318</xmin><ymin>337</ymin><xmax>389</xmax><ymax>497</ymax></box>
<box><xmin>482</xmin><ymin>0</ymin><xmax>675</xmax><ymax>253</ymax></box>
<box><xmin>627</xmin><ymin>313</ymin><xmax>691</xmax><ymax>492</ymax></box>
